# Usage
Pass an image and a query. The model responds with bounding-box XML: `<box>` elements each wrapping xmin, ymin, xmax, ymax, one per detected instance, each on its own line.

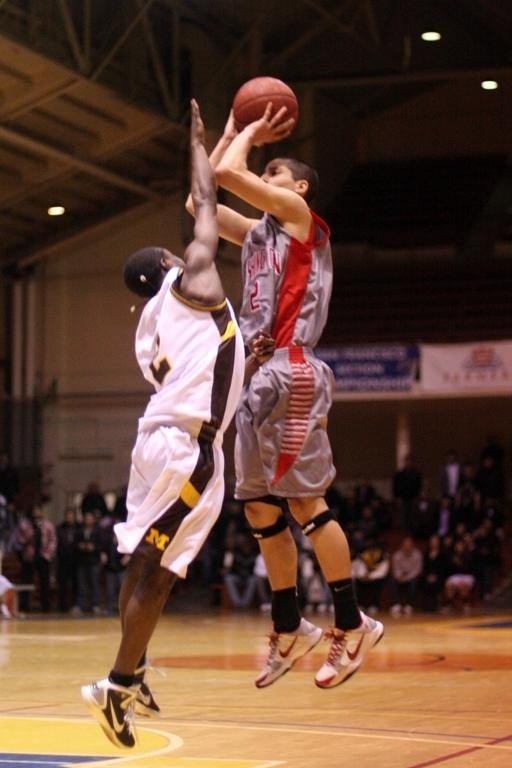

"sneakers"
<box><xmin>82</xmin><ymin>677</ymin><xmax>142</xmax><ymax>747</ymax></box>
<box><xmin>316</xmin><ymin>611</ymin><xmax>384</xmax><ymax>688</ymax></box>
<box><xmin>127</xmin><ymin>657</ymin><xmax>160</xmax><ymax>717</ymax></box>
<box><xmin>255</xmin><ymin>616</ymin><xmax>323</xmax><ymax>689</ymax></box>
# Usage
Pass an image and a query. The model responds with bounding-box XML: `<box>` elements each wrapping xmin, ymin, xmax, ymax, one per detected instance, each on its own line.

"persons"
<box><xmin>2</xmin><ymin>484</ymin><xmax>127</xmax><ymax>619</ymax></box>
<box><xmin>182</xmin><ymin>101</ymin><xmax>388</xmax><ymax>691</ymax></box>
<box><xmin>286</xmin><ymin>512</ymin><xmax>335</xmax><ymax>612</ymax></box>
<box><xmin>331</xmin><ymin>444</ymin><xmax>510</xmax><ymax>614</ymax></box>
<box><xmin>166</xmin><ymin>496</ymin><xmax>274</xmax><ymax>612</ymax></box>
<box><xmin>80</xmin><ymin>97</ymin><xmax>280</xmax><ymax>753</ymax></box>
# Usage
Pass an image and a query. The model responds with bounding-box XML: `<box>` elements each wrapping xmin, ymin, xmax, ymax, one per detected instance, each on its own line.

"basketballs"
<box><xmin>232</xmin><ymin>75</ymin><xmax>298</xmax><ymax>135</ymax></box>
